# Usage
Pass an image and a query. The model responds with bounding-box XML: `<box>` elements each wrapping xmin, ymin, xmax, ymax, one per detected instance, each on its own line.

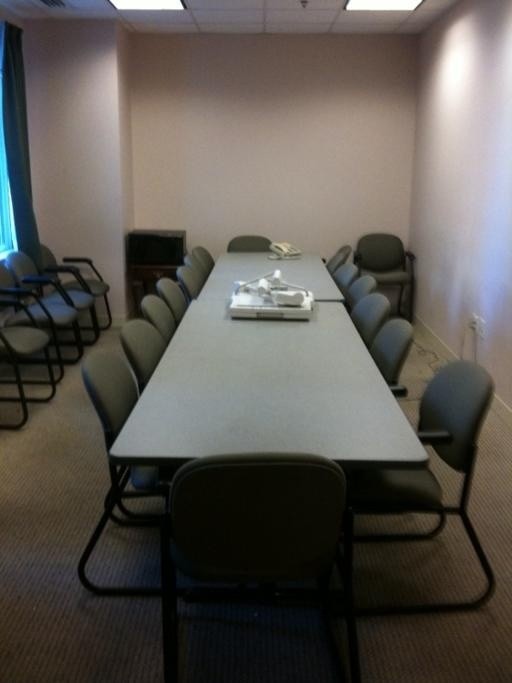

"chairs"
<box><xmin>0</xmin><ymin>244</ymin><xmax>111</xmax><ymax>431</ymax></box>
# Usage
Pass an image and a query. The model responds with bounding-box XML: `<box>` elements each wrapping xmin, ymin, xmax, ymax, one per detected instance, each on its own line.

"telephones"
<box><xmin>268</xmin><ymin>242</ymin><xmax>302</xmax><ymax>260</ymax></box>
